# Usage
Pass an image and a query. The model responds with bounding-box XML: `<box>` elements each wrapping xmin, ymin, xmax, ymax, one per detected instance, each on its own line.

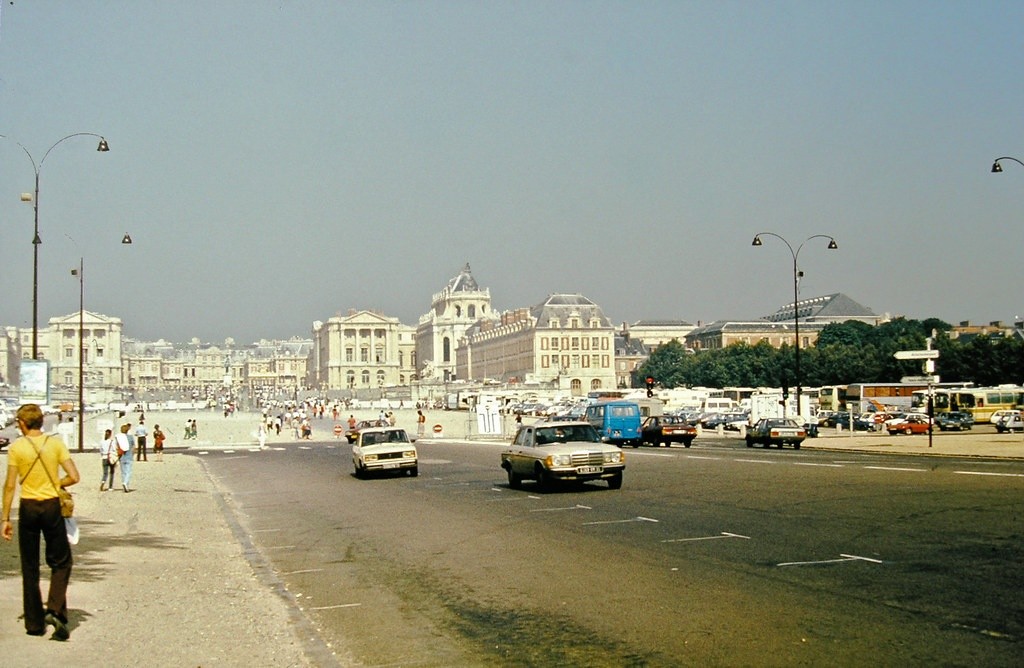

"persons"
<box><xmin>100</xmin><ymin>412</ymin><xmax>165</xmax><ymax>493</ymax></box>
<box><xmin>184</xmin><ymin>385</ymin><xmax>427</xmax><ymax>447</ymax></box>
<box><xmin>515</xmin><ymin>414</ymin><xmax>522</xmax><ymax>427</ymax></box>
<box><xmin>2</xmin><ymin>404</ymin><xmax>80</xmax><ymax>640</ymax></box>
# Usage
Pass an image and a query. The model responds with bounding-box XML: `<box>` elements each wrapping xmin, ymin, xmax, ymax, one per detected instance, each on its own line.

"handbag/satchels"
<box><xmin>59</xmin><ymin>488</ymin><xmax>74</xmax><ymax>517</ymax></box>
<box><xmin>108</xmin><ymin>452</ymin><xmax>119</xmax><ymax>464</ymax></box>
<box><xmin>115</xmin><ymin>436</ymin><xmax>124</xmax><ymax>456</ymax></box>
<box><xmin>64</xmin><ymin>518</ymin><xmax>80</xmax><ymax>544</ymax></box>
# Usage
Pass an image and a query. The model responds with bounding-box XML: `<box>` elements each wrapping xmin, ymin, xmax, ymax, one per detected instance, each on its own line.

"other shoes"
<box><xmin>100</xmin><ymin>481</ymin><xmax>105</xmax><ymax>490</ymax></box>
<box><xmin>109</xmin><ymin>488</ymin><xmax>114</xmax><ymax>491</ymax></box>
<box><xmin>25</xmin><ymin>617</ymin><xmax>41</xmax><ymax>631</ymax></box>
<box><xmin>45</xmin><ymin>609</ymin><xmax>69</xmax><ymax>639</ymax></box>
<box><xmin>122</xmin><ymin>483</ymin><xmax>129</xmax><ymax>492</ymax></box>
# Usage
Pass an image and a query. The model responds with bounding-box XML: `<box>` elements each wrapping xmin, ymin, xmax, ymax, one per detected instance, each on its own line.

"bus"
<box><xmin>411</xmin><ymin>381</ymin><xmax>977</xmax><ymax>413</ymax></box>
<box><xmin>909</xmin><ymin>384</ymin><xmax>1024</xmax><ymax>423</ymax></box>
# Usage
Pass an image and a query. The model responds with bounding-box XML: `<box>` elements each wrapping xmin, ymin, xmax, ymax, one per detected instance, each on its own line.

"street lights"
<box><xmin>752</xmin><ymin>232</ymin><xmax>838</xmax><ymax>416</ymax></box>
<box><xmin>0</xmin><ymin>131</ymin><xmax>109</xmax><ymax>360</ymax></box>
<box><xmin>30</xmin><ymin>230</ymin><xmax>133</xmax><ymax>452</ymax></box>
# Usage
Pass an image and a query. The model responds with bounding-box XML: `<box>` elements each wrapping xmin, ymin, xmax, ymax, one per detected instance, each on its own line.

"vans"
<box><xmin>581</xmin><ymin>402</ymin><xmax>642</xmax><ymax>449</ymax></box>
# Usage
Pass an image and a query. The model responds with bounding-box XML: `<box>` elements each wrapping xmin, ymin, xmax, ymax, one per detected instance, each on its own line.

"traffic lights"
<box><xmin>645</xmin><ymin>375</ymin><xmax>654</xmax><ymax>399</ymax></box>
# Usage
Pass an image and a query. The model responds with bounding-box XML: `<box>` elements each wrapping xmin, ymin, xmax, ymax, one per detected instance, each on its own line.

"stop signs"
<box><xmin>432</xmin><ymin>424</ymin><xmax>443</xmax><ymax>433</ymax></box>
<box><xmin>334</xmin><ymin>425</ymin><xmax>342</xmax><ymax>434</ymax></box>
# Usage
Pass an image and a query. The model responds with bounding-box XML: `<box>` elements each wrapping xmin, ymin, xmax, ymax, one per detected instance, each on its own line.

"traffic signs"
<box><xmin>900</xmin><ymin>375</ymin><xmax>940</xmax><ymax>384</ymax></box>
<box><xmin>893</xmin><ymin>349</ymin><xmax>940</xmax><ymax>360</ymax></box>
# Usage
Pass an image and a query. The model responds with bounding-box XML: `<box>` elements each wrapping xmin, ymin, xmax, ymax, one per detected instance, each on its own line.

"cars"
<box><xmin>498</xmin><ymin>401</ymin><xmax>587</xmax><ymax>422</ymax></box>
<box><xmin>345</xmin><ymin>419</ymin><xmax>389</xmax><ymax>445</ymax></box>
<box><xmin>501</xmin><ymin>421</ymin><xmax>627</xmax><ymax>490</ymax></box>
<box><xmin>639</xmin><ymin>416</ymin><xmax>698</xmax><ymax>448</ymax></box>
<box><xmin>0</xmin><ymin>397</ymin><xmax>109</xmax><ymax>453</ymax></box>
<box><xmin>350</xmin><ymin>426</ymin><xmax>418</xmax><ymax>480</ymax></box>
<box><xmin>815</xmin><ymin>409</ymin><xmax>975</xmax><ymax>436</ymax></box>
<box><xmin>989</xmin><ymin>409</ymin><xmax>1024</xmax><ymax>435</ymax></box>
<box><xmin>744</xmin><ymin>417</ymin><xmax>806</xmax><ymax>450</ymax></box>
<box><xmin>660</xmin><ymin>409</ymin><xmax>752</xmax><ymax>430</ymax></box>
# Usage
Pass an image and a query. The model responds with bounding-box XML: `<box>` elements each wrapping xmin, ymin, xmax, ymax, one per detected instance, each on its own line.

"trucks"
<box><xmin>750</xmin><ymin>393</ymin><xmax>819</xmax><ymax>439</ymax></box>
